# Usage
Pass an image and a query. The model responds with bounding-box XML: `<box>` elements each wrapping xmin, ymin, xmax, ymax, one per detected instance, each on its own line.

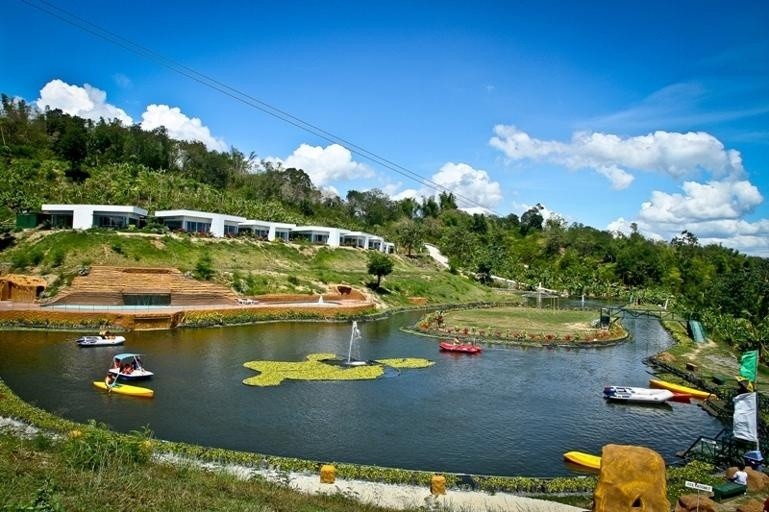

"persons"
<box><xmin>101</xmin><ymin>330</ymin><xmax>115</xmax><ymax>339</ymax></box>
<box><xmin>104</xmin><ymin>372</ymin><xmax>119</xmax><ymax>389</ymax></box>
<box><xmin>727</xmin><ymin>461</ymin><xmax>749</xmax><ymax>486</ymax></box>
<box><xmin>121</xmin><ymin>363</ymin><xmax>134</xmax><ymax>373</ymax></box>
<box><xmin>111</xmin><ymin>358</ymin><xmax>124</xmax><ymax>373</ymax></box>
<box><xmin>131</xmin><ymin>357</ymin><xmax>143</xmax><ymax>370</ymax></box>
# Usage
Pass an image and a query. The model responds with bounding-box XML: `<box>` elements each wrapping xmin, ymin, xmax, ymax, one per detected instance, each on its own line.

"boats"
<box><xmin>439</xmin><ymin>342</ymin><xmax>482</xmax><ymax>353</ymax></box>
<box><xmin>93</xmin><ymin>381</ymin><xmax>154</xmax><ymax>397</ymax></box>
<box><xmin>603</xmin><ymin>379</ymin><xmax>719</xmax><ymax>403</ymax></box>
<box><xmin>75</xmin><ymin>336</ymin><xmax>127</xmax><ymax>346</ymax></box>
<box><xmin>562</xmin><ymin>451</ymin><xmax>602</xmax><ymax>470</ymax></box>
<box><xmin>109</xmin><ymin>353</ymin><xmax>154</xmax><ymax>381</ymax></box>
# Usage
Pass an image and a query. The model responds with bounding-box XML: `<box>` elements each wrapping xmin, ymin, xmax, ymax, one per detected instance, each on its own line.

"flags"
<box><xmin>739</xmin><ymin>349</ymin><xmax>757</xmax><ymax>383</ymax></box>
<box><xmin>731</xmin><ymin>392</ymin><xmax>758</xmax><ymax>443</ymax></box>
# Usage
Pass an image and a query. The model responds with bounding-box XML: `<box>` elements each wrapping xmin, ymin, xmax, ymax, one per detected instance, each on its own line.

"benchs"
<box><xmin>712</xmin><ymin>482</ymin><xmax>746</xmax><ymax>502</ymax></box>
